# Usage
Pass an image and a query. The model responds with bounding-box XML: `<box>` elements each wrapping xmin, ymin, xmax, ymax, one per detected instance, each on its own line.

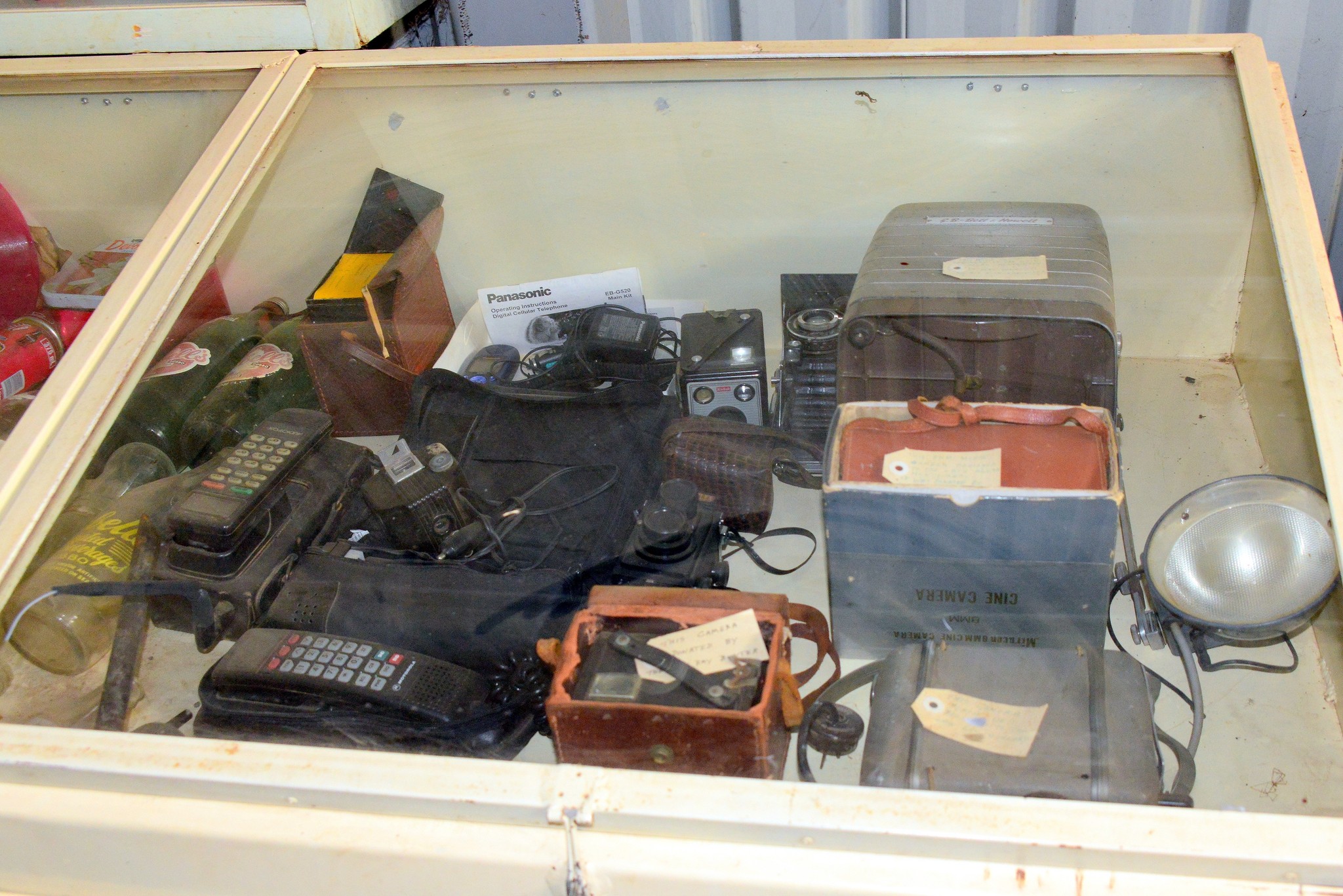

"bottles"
<box><xmin>0</xmin><ymin>297</ymin><xmax>323</xmax><ymax>676</ymax></box>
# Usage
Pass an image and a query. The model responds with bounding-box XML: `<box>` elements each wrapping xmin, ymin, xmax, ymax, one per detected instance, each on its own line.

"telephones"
<box><xmin>192</xmin><ymin>616</ymin><xmax>539</xmax><ymax>762</ymax></box>
<box><xmin>150</xmin><ymin>406</ymin><xmax>375</xmax><ymax>642</ymax></box>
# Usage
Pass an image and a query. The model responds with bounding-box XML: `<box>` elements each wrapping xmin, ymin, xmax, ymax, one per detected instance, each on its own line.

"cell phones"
<box><xmin>465</xmin><ymin>344</ymin><xmax>520</xmax><ymax>389</ymax></box>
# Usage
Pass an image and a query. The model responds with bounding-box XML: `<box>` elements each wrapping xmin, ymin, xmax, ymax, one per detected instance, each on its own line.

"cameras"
<box><xmin>773</xmin><ymin>273</ymin><xmax>856</xmax><ymax>482</ymax></box>
<box><xmin>606</xmin><ymin>477</ymin><xmax>732</xmax><ymax>592</ymax></box>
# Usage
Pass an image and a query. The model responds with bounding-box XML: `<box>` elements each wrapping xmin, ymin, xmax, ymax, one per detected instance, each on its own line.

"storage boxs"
<box><xmin>810</xmin><ymin>392</ymin><xmax>1134</xmax><ymax>658</ymax></box>
<box><xmin>296</xmin><ymin>202</ymin><xmax>458</xmax><ymax>442</ymax></box>
<box><xmin>535</xmin><ymin>580</ymin><xmax>806</xmax><ymax>781</ymax></box>
